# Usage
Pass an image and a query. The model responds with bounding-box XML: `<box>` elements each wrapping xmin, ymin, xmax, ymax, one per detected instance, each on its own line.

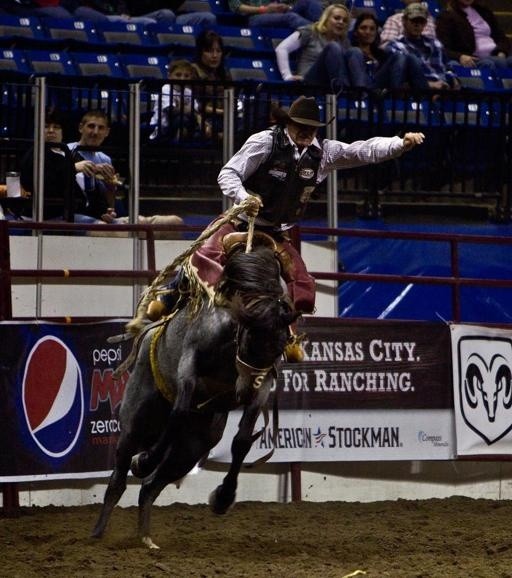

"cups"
<box><xmin>6</xmin><ymin>171</ymin><xmax>21</xmax><ymax>198</ymax></box>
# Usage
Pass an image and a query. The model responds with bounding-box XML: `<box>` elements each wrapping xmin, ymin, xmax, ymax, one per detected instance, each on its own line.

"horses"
<box><xmin>87</xmin><ymin>246</ymin><xmax>303</xmax><ymax>550</ymax></box>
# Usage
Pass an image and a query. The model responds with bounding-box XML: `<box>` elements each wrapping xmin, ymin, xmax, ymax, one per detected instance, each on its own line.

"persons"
<box><xmin>1</xmin><ymin>107</ymin><xmax>184</xmax><ymax>224</ymax></box>
<box><xmin>148</xmin><ymin>29</ymin><xmax>246</xmax><ymax>138</ymax></box>
<box><xmin>229</xmin><ymin>1</ymin><xmax>510</xmax><ymax>102</ymax></box>
<box><xmin>122</xmin><ymin>94</ymin><xmax>427</xmax><ymax>363</ymax></box>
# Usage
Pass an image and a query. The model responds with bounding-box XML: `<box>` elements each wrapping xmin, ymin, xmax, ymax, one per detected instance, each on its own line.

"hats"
<box><xmin>403</xmin><ymin>2</ymin><xmax>428</xmax><ymax>23</ymax></box>
<box><xmin>272</xmin><ymin>95</ymin><xmax>335</xmax><ymax>127</ymax></box>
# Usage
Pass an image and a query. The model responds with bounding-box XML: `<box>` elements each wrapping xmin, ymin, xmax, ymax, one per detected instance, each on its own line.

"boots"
<box><xmin>123</xmin><ymin>300</ymin><xmax>167</xmax><ymax>334</ymax></box>
<box><xmin>285</xmin><ymin>343</ymin><xmax>306</xmax><ymax>363</ymax></box>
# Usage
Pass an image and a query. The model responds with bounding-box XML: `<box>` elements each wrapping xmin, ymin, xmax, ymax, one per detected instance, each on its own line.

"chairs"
<box><xmin>1</xmin><ymin>1</ymin><xmax>512</xmax><ymax>148</ymax></box>
